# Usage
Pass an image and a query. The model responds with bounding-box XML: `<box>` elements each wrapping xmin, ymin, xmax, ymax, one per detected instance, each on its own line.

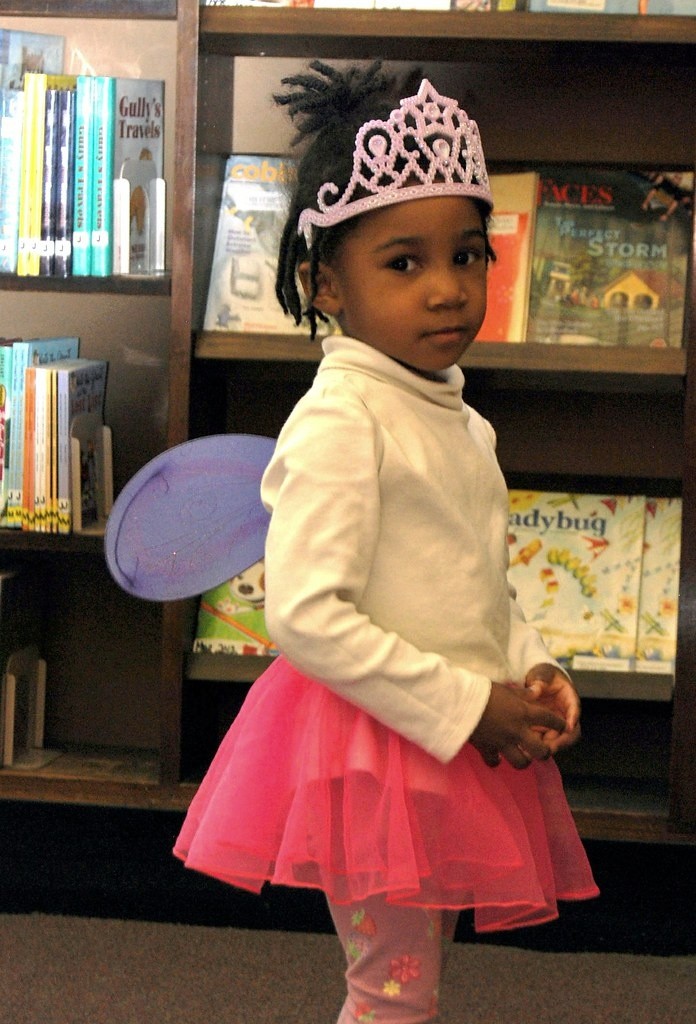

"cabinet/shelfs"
<box><xmin>0</xmin><ymin>0</ymin><xmax>695</xmax><ymax>843</ymax></box>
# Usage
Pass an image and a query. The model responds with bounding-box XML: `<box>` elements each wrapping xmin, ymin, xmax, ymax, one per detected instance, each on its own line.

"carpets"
<box><xmin>0</xmin><ymin>912</ymin><xmax>696</xmax><ymax>1023</ymax></box>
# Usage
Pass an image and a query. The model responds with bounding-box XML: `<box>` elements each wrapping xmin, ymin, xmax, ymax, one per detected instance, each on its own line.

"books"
<box><xmin>200</xmin><ymin>0</ymin><xmax>695</xmax><ymax>19</ymax></box>
<box><xmin>0</xmin><ymin>27</ymin><xmax>166</xmax><ymax>282</ymax></box>
<box><xmin>0</xmin><ymin>337</ymin><xmax>112</xmax><ymax>534</ymax></box>
<box><xmin>191</xmin><ymin>491</ymin><xmax>684</xmax><ymax>680</ymax></box>
<box><xmin>202</xmin><ymin>151</ymin><xmax>696</xmax><ymax>352</ymax></box>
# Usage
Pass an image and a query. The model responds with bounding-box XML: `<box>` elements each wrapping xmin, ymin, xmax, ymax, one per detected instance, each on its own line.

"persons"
<box><xmin>171</xmin><ymin>58</ymin><xmax>603</xmax><ymax>1024</ymax></box>
